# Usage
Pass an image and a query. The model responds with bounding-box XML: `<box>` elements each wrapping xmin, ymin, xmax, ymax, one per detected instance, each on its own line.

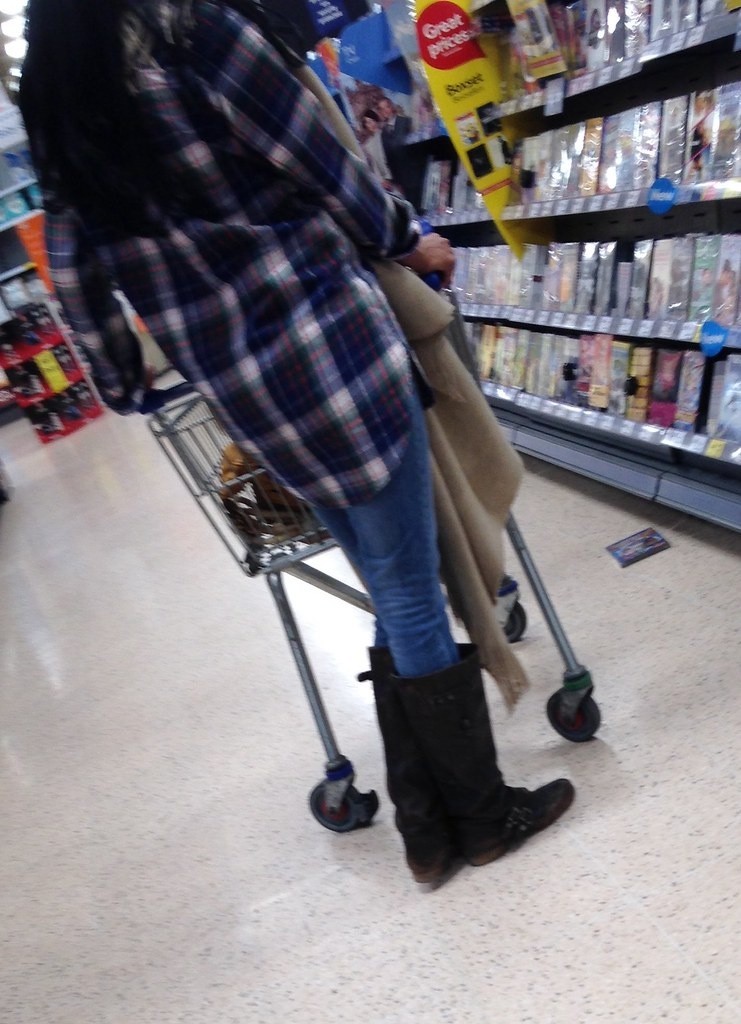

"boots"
<box><xmin>388</xmin><ymin>643</ymin><xmax>577</xmax><ymax>866</ymax></box>
<box><xmin>358</xmin><ymin>643</ymin><xmax>462</xmax><ymax>892</ymax></box>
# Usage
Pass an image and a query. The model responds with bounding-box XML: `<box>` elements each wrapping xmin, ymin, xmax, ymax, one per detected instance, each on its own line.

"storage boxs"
<box><xmin>0</xmin><ymin>302</ymin><xmax>123</xmax><ymax>445</ymax></box>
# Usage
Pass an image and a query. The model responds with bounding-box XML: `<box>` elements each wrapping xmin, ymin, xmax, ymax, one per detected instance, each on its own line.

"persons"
<box><xmin>361</xmin><ymin>97</ymin><xmax>428</xmax><ymax>216</ymax></box>
<box><xmin>16</xmin><ymin>0</ymin><xmax>576</xmax><ymax>882</ymax></box>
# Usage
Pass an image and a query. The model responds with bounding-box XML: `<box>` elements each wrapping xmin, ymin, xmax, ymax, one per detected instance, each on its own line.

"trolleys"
<box><xmin>141</xmin><ymin>359</ymin><xmax>602</xmax><ymax>835</ymax></box>
